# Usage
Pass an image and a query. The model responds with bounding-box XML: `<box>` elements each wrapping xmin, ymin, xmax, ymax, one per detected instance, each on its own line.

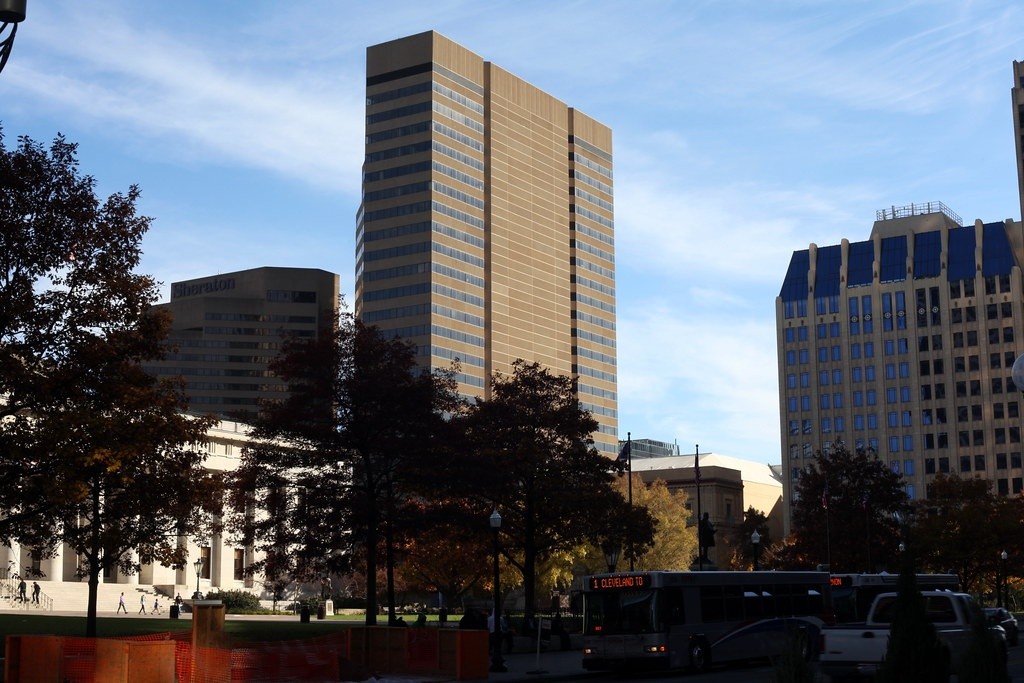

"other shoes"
<box><xmin>117</xmin><ymin>612</ymin><xmax>128</xmax><ymax>614</ymax></box>
<box><xmin>178</xmin><ymin>613</ymin><xmax>182</xmax><ymax>615</ymax></box>
<box><xmin>138</xmin><ymin>612</ymin><xmax>147</xmax><ymax>615</ymax></box>
<box><xmin>151</xmin><ymin>613</ymin><xmax>161</xmax><ymax>615</ymax></box>
<box><xmin>19</xmin><ymin>600</ymin><xmax>40</xmax><ymax>605</ymax></box>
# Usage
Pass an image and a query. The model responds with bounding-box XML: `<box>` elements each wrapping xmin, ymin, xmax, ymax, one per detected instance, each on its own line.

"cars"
<box><xmin>983</xmin><ymin>606</ymin><xmax>1023</xmax><ymax>645</ymax></box>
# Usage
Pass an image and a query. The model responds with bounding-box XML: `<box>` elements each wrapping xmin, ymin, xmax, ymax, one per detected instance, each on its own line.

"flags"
<box><xmin>822</xmin><ymin>485</ymin><xmax>828</xmax><ymax>509</ymax></box>
<box><xmin>694</xmin><ymin>457</ymin><xmax>701</xmax><ymax>486</ymax></box>
<box><xmin>862</xmin><ymin>494</ymin><xmax>869</xmax><ymax>510</ymax></box>
<box><xmin>616</xmin><ymin>442</ymin><xmax>629</xmax><ymax>462</ymax></box>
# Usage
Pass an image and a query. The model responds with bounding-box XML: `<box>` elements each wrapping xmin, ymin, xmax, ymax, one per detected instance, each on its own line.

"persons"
<box><xmin>487</xmin><ymin>608</ymin><xmax>515</xmax><ymax>654</ymax></box>
<box><xmin>32</xmin><ymin>582</ymin><xmax>40</xmax><ymax>604</ymax></box>
<box><xmin>139</xmin><ymin>595</ymin><xmax>146</xmax><ymax>615</ymax></box>
<box><xmin>551</xmin><ymin>613</ymin><xmax>572</xmax><ymax>651</ymax></box>
<box><xmin>412</xmin><ymin>614</ymin><xmax>426</xmax><ymax>627</ymax></box>
<box><xmin>151</xmin><ymin>599</ymin><xmax>162</xmax><ymax>615</ymax></box>
<box><xmin>18</xmin><ymin>579</ymin><xmax>26</xmax><ymax>603</ymax></box>
<box><xmin>175</xmin><ymin>593</ymin><xmax>184</xmax><ymax>614</ymax></box>
<box><xmin>701</xmin><ymin>512</ymin><xmax>718</xmax><ymax>556</ymax></box>
<box><xmin>117</xmin><ymin>592</ymin><xmax>128</xmax><ymax>614</ymax></box>
<box><xmin>521</xmin><ymin>611</ymin><xmax>551</xmax><ymax>647</ymax></box>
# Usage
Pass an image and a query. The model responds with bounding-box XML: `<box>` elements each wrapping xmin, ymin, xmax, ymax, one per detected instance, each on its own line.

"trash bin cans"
<box><xmin>170</xmin><ymin>605</ymin><xmax>179</xmax><ymax>619</ymax></box>
<box><xmin>300</xmin><ymin>605</ymin><xmax>310</xmax><ymax>623</ymax></box>
<box><xmin>439</xmin><ymin>608</ymin><xmax>447</xmax><ymax>622</ymax></box>
<box><xmin>317</xmin><ymin>604</ymin><xmax>326</xmax><ymax>619</ymax></box>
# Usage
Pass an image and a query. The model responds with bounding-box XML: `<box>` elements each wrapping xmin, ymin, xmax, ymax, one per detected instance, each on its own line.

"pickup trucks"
<box><xmin>818</xmin><ymin>588</ymin><xmax>1010</xmax><ymax>683</ymax></box>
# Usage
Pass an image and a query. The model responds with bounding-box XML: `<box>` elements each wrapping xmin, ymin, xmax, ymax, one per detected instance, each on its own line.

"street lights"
<box><xmin>750</xmin><ymin>530</ymin><xmax>761</xmax><ymax>572</ymax></box>
<box><xmin>1000</xmin><ymin>549</ymin><xmax>1011</xmax><ymax>611</ymax></box>
<box><xmin>487</xmin><ymin>508</ymin><xmax>508</xmax><ymax>672</ymax></box>
<box><xmin>191</xmin><ymin>558</ymin><xmax>203</xmax><ymax>600</ymax></box>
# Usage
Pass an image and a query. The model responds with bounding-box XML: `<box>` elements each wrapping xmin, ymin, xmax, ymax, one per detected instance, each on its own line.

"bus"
<box><xmin>826</xmin><ymin>571</ymin><xmax>960</xmax><ymax>625</ymax></box>
<box><xmin>569</xmin><ymin>563</ymin><xmax>838</xmax><ymax>677</ymax></box>
